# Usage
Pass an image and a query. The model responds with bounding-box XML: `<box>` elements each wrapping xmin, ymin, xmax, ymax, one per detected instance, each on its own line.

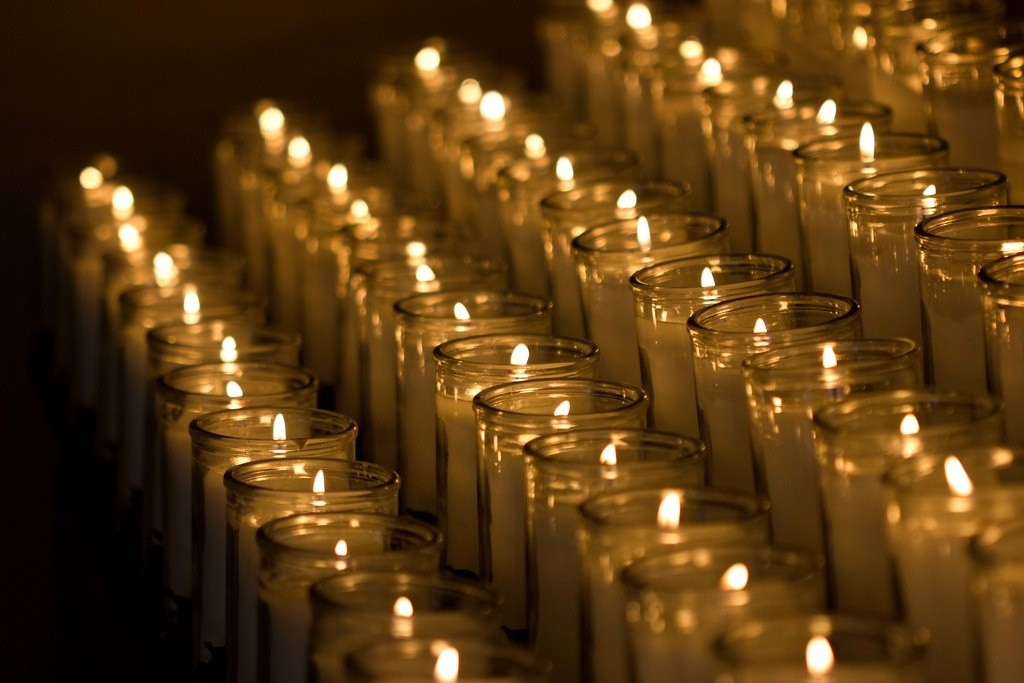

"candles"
<box><xmin>54</xmin><ymin>3</ymin><xmax>1023</xmax><ymax>682</ymax></box>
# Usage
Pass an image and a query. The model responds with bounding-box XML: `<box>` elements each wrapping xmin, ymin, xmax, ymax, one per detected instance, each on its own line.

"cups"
<box><xmin>69</xmin><ymin>0</ymin><xmax>1024</xmax><ymax>683</ymax></box>
